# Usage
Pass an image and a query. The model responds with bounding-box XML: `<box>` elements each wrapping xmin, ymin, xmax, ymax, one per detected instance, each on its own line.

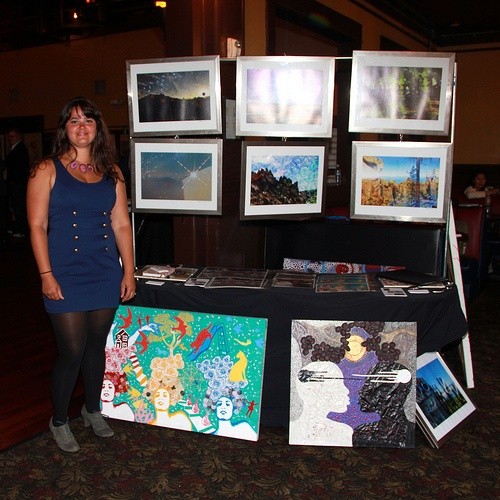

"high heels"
<box><xmin>49</xmin><ymin>416</ymin><xmax>80</xmax><ymax>453</ymax></box>
<box><xmin>81</xmin><ymin>403</ymin><xmax>114</xmax><ymax>438</ymax></box>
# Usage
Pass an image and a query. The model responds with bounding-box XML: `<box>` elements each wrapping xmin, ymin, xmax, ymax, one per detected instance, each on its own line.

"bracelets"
<box><xmin>38</xmin><ymin>270</ymin><xmax>53</xmax><ymax>275</ymax></box>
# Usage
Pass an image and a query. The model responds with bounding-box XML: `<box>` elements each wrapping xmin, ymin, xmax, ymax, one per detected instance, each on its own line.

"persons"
<box><xmin>463</xmin><ymin>171</ymin><xmax>500</xmax><ymax>275</ymax></box>
<box><xmin>26</xmin><ymin>98</ymin><xmax>136</xmax><ymax>453</ymax></box>
<box><xmin>0</xmin><ymin>126</ymin><xmax>29</xmax><ymax>237</ymax></box>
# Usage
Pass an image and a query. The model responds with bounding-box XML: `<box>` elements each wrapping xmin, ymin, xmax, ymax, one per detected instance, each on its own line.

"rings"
<box><xmin>134</xmin><ymin>292</ymin><xmax>136</xmax><ymax>295</ymax></box>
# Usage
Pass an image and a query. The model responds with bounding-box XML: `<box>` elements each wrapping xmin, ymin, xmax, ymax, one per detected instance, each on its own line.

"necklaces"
<box><xmin>69</xmin><ymin>153</ymin><xmax>94</xmax><ymax>172</ymax></box>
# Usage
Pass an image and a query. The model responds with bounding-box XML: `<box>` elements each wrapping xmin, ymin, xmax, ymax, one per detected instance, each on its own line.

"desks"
<box><xmin>122</xmin><ymin>279</ymin><xmax>469</xmax><ymax>432</ymax></box>
<box><xmin>453</xmin><ymin>204</ymin><xmax>492</xmax><ymax>279</ymax></box>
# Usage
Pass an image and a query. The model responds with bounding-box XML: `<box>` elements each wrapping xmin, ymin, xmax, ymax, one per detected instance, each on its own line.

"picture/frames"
<box><xmin>236</xmin><ymin>56</ymin><xmax>335</xmax><ymax>138</ymax></box>
<box><xmin>239</xmin><ymin>140</ymin><xmax>329</xmax><ymax>221</ymax></box>
<box><xmin>130</xmin><ymin>137</ymin><xmax>222</xmax><ymax>215</ymax></box>
<box><xmin>350</xmin><ymin>140</ymin><xmax>453</xmax><ymax>224</ymax></box>
<box><xmin>348</xmin><ymin>50</ymin><xmax>455</xmax><ymax>136</ymax></box>
<box><xmin>412</xmin><ymin>348</ymin><xmax>480</xmax><ymax>449</ymax></box>
<box><xmin>126</xmin><ymin>54</ymin><xmax>223</xmax><ymax>137</ymax></box>
